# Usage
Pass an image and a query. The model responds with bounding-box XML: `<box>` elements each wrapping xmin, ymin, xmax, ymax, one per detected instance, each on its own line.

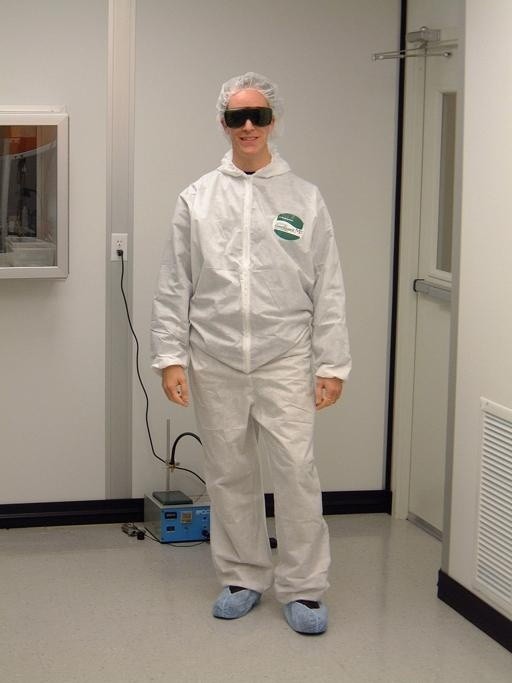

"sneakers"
<box><xmin>212</xmin><ymin>585</ymin><xmax>260</xmax><ymax>619</ymax></box>
<box><xmin>283</xmin><ymin>601</ymin><xmax>327</xmax><ymax>635</ymax></box>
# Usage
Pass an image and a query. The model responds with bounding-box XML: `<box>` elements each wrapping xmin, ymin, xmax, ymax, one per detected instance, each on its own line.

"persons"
<box><xmin>148</xmin><ymin>71</ymin><xmax>354</xmax><ymax>634</ymax></box>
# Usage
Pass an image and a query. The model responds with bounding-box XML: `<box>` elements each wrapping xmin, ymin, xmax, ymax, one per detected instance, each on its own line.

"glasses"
<box><xmin>225</xmin><ymin>107</ymin><xmax>273</xmax><ymax>128</ymax></box>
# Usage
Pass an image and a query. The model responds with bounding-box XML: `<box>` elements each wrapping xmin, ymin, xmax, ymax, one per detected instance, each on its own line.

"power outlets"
<box><xmin>110</xmin><ymin>231</ymin><xmax>129</xmax><ymax>263</ymax></box>
